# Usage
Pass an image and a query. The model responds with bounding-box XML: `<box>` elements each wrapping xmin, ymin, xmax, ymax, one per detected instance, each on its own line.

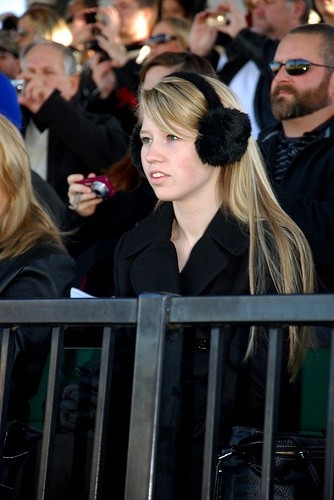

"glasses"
<box><xmin>144</xmin><ymin>34</ymin><xmax>177</xmax><ymax>46</ymax></box>
<box><xmin>267</xmin><ymin>59</ymin><xmax>334</xmax><ymax>80</ymax></box>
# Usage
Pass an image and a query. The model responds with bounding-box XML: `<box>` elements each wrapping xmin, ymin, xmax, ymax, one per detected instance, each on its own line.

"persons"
<box><xmin>257</xmin><ymin>23</ymin><xmax>334</xmax><ymax>295</ymax></box>
<box><xmin>59</xmin><ymin>71</ymin><xmax>315</xmax><ymax>500</ymax></box>
<box><xmin>0</xmin><ymin>113</ymin><xmax>79</xmax><ymax>500</ymax></box>
<box><xmin>19</xmin><ymin>39</ymin><xmax>127</xmax><ymax>204</ymax></box>
<box><xmin>67</xmin><ymin>51</ymin><xmax>218</xmax><ymax>297</ymax></box>
<box><xmin>0</xmin><ymin>0</ymin><xmax>334</xmax><ymax>136</ymax></box>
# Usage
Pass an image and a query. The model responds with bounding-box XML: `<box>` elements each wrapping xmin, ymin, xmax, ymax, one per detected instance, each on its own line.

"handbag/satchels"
<box><xmin>213</xmin><ymin>425</ymin><xmax>326</xmax><ymax>500</ymax></box>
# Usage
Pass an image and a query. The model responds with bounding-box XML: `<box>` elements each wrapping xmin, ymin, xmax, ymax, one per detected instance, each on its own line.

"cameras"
<box><xmin>85</xmin><ymin>10</ymin><xmax>102</xmax><ymax>24</ymax></box>
<box><xmin>10</xmin><ymin>79</ymin><xmax>25</xmax><ymax>97</ymax></box>
<box><xmin>73</xmin><ymin>176</ymin><xmax>116</xmax><ymax>199</ymax></box>
<box><xmin>204</xmin><ymin>9</ymin><xmax>232</xmax><ymax>28</ymax></box>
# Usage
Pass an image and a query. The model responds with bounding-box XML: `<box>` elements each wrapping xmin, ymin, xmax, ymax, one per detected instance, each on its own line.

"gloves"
<box><xmin>58</xmin><ymin>384</ymin><xmax>79</xmax><ymax>430</ymax></box>
<box><xmin>68</xmin><ymin>374</ymin><xmax>98</xmax><ymax>423</ymax></box>
